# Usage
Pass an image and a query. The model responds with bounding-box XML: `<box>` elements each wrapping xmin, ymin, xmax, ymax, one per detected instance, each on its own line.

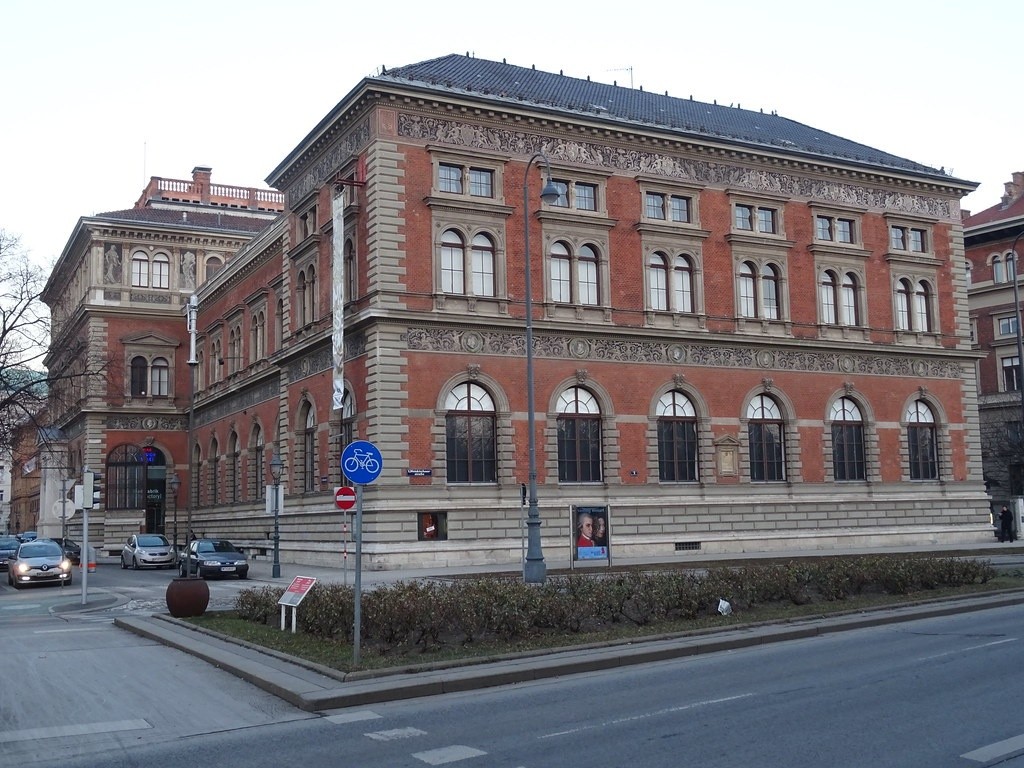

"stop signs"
<box><xmin>334</xmin><ymin>487</ymin><xmax>356</xmax><ymax>510</ymax></box>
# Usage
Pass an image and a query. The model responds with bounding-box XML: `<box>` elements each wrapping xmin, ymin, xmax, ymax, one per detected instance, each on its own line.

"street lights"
<box><xmin>522</xmin><ymin>152</ymin><xmax>562</xmax><ymax>584</ymax></box>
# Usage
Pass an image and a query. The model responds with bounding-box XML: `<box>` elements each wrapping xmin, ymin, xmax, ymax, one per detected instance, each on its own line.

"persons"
<box><xmin>593</xmin><ymin>516</ymin><xmax>606</xmax><ymax>546</ymax></box>
<box><xmin>577</xmin><ymin>513</ymin><xmax>594</xmax><ymax>547</ymax></box>
<box><xmin>997</xmin><ymin>506</ymin><xmax>1013</xmax><ymax>543</ymax></box>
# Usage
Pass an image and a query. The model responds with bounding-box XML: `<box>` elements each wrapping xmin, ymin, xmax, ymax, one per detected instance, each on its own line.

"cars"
<box><xmin>178</xmin><ymin>538</ymin><xmax>250</xmax><ymax>580</ymax></box>
<box><xmin>6</xmin><ymin>542</ymin><xmax>72</xmax><ymax>589</ymax></box>
<box><xmin>0</xmin><ymin>538</ymin><xmax>22</xmax><ymax>569</ymax></box>
<box><xmin>120</xmin><ymin>534</ymin><xmax>177</xmax><ymax>570</ymax></box>
<box><xmin>31</xmin><ymin>537</ymin><xmax>81</xmax><ymax>565</ymax></box>
<box><xmin>15</xmin><ymin>531</ymin><xmax>37</xmax><ymax>544</ymax></box>
